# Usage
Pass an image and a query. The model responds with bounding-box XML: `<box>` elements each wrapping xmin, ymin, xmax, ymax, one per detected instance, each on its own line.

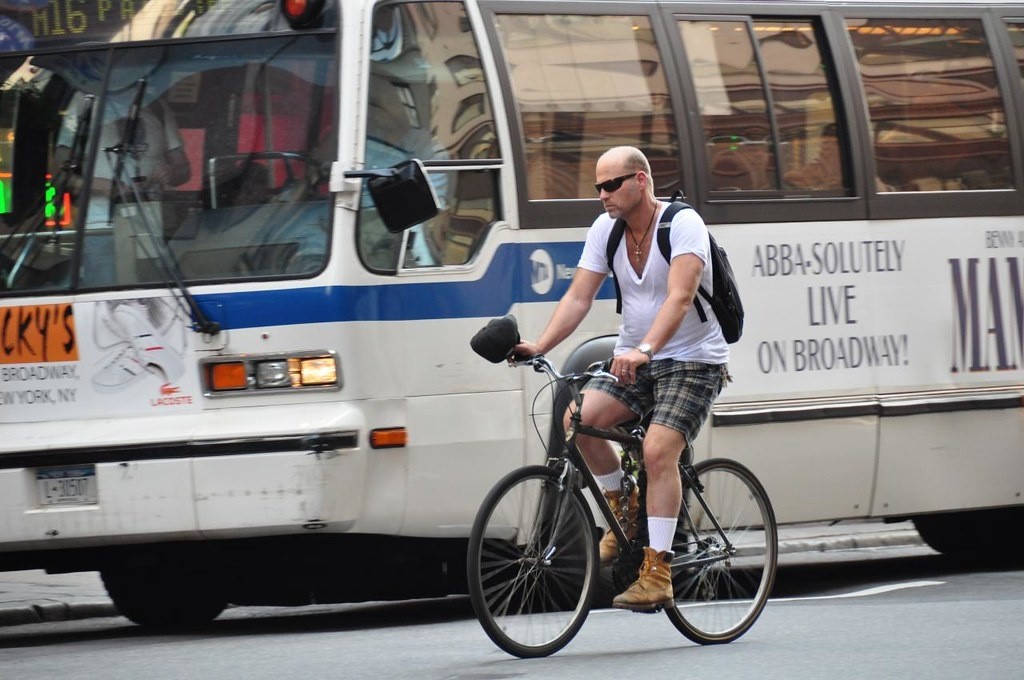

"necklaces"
<box><xmin>628</xmin><ymin>201</ymin><xmax>657</xmax><ymax>263</ymax></box>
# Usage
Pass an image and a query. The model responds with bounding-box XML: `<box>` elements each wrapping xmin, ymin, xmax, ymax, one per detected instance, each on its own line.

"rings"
<box><xmin>622</xmin><ymin>370</ymin><xmax>627</xmax><ymax>373</ymax></box>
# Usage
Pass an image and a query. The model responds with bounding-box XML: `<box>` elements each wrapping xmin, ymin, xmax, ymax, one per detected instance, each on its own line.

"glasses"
<box><xmin>595</xmin><ymin>173</ymin><xmax>637</xmax><ymax>193</ymax></box>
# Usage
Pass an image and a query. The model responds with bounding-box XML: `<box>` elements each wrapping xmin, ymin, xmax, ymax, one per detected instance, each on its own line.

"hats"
<box><xmin>470</xmin><ymin>314</ymin><xmax>520</xmax><ymax>363</ymax></box>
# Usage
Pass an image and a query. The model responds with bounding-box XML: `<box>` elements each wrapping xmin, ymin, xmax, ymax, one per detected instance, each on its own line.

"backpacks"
<box><xmin>606</xmin><ymin>189</ymin><xmax>744</xmax><ymax>344</ymax></box>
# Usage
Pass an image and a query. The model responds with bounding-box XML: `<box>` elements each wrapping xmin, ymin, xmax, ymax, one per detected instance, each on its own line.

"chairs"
<box><xmin>525</xmin><ymin>122</ymin><xmax>858</xmax><ymax>202</ymax></box>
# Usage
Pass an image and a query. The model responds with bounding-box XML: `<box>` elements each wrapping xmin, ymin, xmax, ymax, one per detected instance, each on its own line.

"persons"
<box><xmin>303</xmin><ymin>72</ymin><xmax>459</xmax><ymax>268</ymax></box>
<box><xmin>53</xmin><ymin>84</ymin><xmax>191</xmax><ymax>287</ymax></box>
<box><xmin>507</xmin><ymin>146</ymin><xmax>731</xmax><ymax>610</ymax></box>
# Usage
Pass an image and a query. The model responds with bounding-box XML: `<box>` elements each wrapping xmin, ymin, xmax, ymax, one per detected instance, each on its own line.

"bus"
<box><xmin>0</xmin><ymin>0</ymin><xmax>1023</xmax><ymax>632</ymax></box>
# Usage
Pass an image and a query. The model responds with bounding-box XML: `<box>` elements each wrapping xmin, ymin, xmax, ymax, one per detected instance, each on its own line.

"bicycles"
<box><xmin>465</xmin><ymin>350</ymin><xmax>778</xmax><ymax>659</ymax></box>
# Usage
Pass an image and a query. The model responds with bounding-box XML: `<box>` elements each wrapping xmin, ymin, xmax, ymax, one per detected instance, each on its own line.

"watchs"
<box><xmin>636</xmin><ymin>343</ymin><xmax>655</xmax><ymax>359</ymax></box>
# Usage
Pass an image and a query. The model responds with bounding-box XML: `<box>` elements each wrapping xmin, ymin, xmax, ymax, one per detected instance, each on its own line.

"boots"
<box><xmin>613</xmin><ymin>545</ymin><xmax>675</xmax><ymax>610</ymax></box>
<box><xmin>598</xmin><ymin>485</ymin><xmax>640</xmax><ymax>568</ymax></box>
